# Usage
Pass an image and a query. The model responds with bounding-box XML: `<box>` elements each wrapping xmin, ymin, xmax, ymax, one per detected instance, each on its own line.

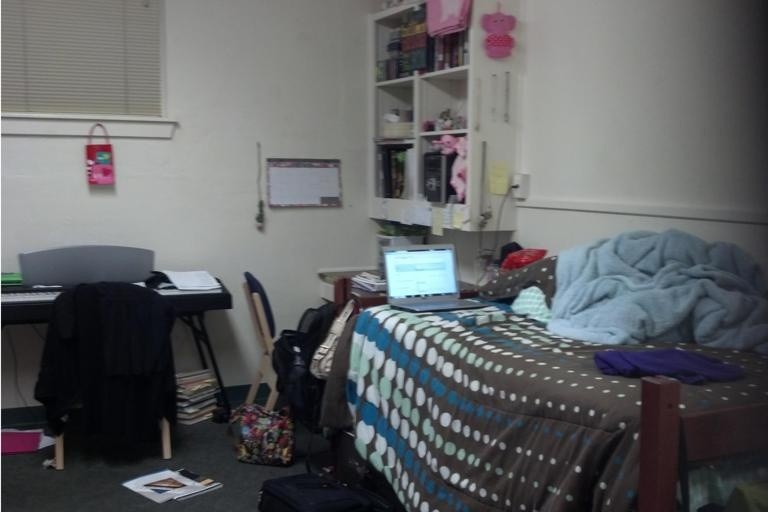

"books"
<box><xmin>427</xmin><ymin>32</ymin><xmax>471</xmax><ymax>72</ymax></box>
<box><xmin>148</xmin><ymin>269</ymin><xmax>222</xmax><ymax>291</ymax></box>
<box><xmin>173</xmin><ymin>467</ymin><xmax>224</xmax><ymax>502</ymax></box>
<box><xmin>1</xmin><ymin>272</ymin><xmax>23</xmax><ymax>286</ymax></box>
<box><xmin>121</xmin><ymin>468</ymin><xmax>206</xmax><ymax>505</ymax></box>
<box><xmin>176</xmin><ymin>365</ymin><xmax>220</xmax><ymax>427</ymax></box>
<box><xmin>375</xmin><ymin>148</ymin><xmax>412</xmax><ymax>200</ymax></box>
<box><xmin>350</xmin><ymin>270</ymin><xmax>386</xmax><ymax>293</ymax></box>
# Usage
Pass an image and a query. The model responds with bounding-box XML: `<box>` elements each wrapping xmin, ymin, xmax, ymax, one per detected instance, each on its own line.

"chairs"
<box><xmin>34</xmin><ymin>280</ymin><xmax>177</xmax><ymax>470</ymax></box>
<box><xmin>17</xmin><ymin>246</ymin><xmax>155</xmax><ymax>285</ymax></box>
<box><xmin>243</xmin><ymin>271</ymin><xmax>278</xmax><ymax>413</ymax></box>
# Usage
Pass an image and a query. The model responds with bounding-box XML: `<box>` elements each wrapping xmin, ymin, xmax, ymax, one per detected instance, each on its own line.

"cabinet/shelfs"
<box><xmin>365</xmin><ymin>1</ymin><xmax>514</xmax><ymax>231</ymax></box>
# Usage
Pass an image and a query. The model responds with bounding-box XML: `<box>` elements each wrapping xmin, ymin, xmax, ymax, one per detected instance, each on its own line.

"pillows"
<box><xmin>476</xmin><ymin>254</ymin><xmax>556</xmax><ymax>304</ymax></box>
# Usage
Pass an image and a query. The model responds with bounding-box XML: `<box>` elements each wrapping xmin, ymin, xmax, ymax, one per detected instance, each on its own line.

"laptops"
<box><xmin>381</xmin><ymin>243</ymin><xmax>490</xmax><ymax>313</ymax></box>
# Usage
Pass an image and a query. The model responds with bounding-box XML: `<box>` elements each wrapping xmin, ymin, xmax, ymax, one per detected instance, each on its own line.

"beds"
<box><xmin>319</xmin><ymin>299</ymin><xmax>767</xmax><ymax>512</ymax></box>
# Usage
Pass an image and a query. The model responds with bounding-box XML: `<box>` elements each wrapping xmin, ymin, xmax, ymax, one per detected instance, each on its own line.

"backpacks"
<box><xmin>271</xmin><ymin>327</ymin><xmax>314</xmax><ymax>414</ymax></box>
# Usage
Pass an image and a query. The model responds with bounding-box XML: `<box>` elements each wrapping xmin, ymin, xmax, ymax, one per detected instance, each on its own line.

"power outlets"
<box><xmin>511</xmin><ymin>173</ymin><xmax>530</xmax><ymax>199</ymax></box>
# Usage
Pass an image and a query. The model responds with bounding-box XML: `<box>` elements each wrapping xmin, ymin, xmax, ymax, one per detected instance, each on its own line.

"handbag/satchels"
<box><xmin>85</xmin><ymin>123</ymin><xmax>116</xmax><ymax>187</ymax></box>
<box><xmin>228</xmin><ymin>403</ymin><xmax>295</xmax><ymax>467</ymax></box>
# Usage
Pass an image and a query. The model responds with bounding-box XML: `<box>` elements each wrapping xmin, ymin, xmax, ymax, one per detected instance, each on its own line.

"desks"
<box><xmin>1</xmin><ymin>272</ymin><xmax>234</xmax><ymax>426</ymax></box>
<box><xmin>319</xmin><ymin>270</ymin><xmax>481</xmax><ymax>308</ymax></box>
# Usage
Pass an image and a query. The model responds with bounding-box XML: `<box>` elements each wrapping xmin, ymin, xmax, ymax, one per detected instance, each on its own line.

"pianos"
<box><xmin>1</xmin><ymin>270</ymin><xmax>232</xmax><ymax>325</ymax></box>
